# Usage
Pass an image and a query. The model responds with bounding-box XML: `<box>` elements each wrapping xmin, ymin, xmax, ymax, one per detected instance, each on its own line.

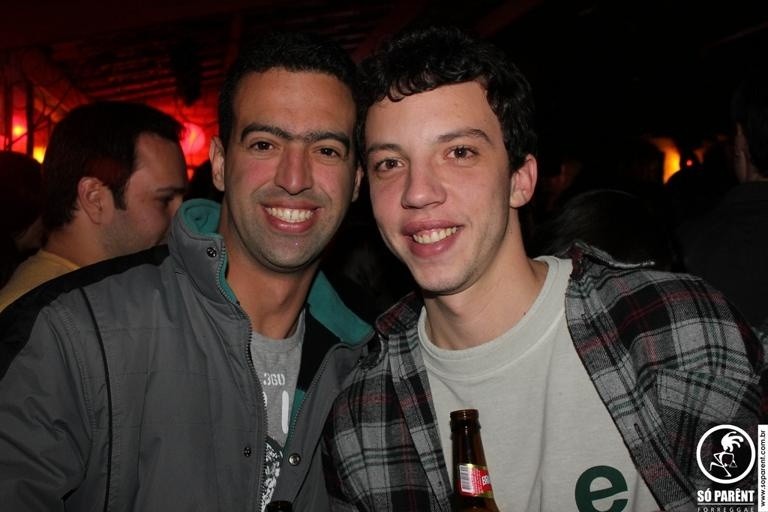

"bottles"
<box><xmin>449</xmin><ymin>408</ymin><xmax>500</xmax><ymax>512</ymax></box>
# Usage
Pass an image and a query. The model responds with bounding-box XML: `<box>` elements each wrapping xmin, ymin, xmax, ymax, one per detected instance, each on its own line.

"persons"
<box><xmin>674</xmin><ymin>76</ymin><xmax>768</xmax><ymax>323</ymax></box>
<box><xmin>0</xmin><ymin>30</ymin><xmax>374</xmax><ymax>512</ymax></box>
<box><xmin>519</xmin><ymin>112</ymin><xmax>731</xmax><ymax>266</ymax></box>
<box><xmin>319</xmin><ymin>26</ymin><xmax>767</xmax><ymax>512</ymax></box>
<box><xmin>0</xmin><ymin>151</ymin><xmax>43</xmax><ymax>285</ymax></box>
<box><xmin>0</xmin><ymin>102</ymin><xmax>188</xmax><ymax>311</ymax></box>
